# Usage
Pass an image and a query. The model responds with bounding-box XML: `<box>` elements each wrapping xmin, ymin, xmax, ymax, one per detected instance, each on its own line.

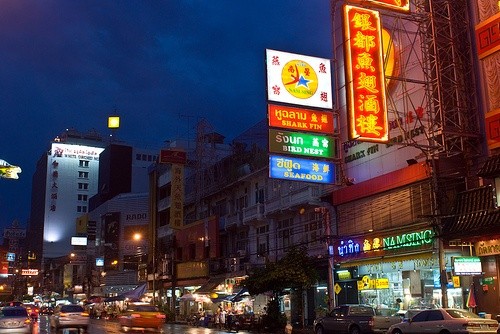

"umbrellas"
<box><xmin>466</xmin><ymin>286</ymin><xmax>478</xmax><ymax>313</ymax></box>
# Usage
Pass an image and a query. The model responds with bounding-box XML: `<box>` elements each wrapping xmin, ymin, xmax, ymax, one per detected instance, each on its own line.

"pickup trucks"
<box><xmin>314</xmin><ymin>304</ymin><xmax>425</xmax><ymax>334</ymax></box>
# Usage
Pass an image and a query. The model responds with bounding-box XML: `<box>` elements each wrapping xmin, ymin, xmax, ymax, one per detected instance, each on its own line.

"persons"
<box><xmin>396</xmin><ymin>298</ymin><xmax>403</xmax><ymax>311</ymax></box>
<box><xmin>214</xmin><ymin>304</ymin><xmax>227</xmax><ymax>330</ymax></box>
<box><xmin>30</xmin><ymin>312</ymin><xmax>39</xmax><ymax>334</ymax></box>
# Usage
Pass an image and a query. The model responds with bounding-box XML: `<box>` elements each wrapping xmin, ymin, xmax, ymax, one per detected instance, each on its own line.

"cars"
<box><xmin>2</xmin><ymin>301</ymin><xmax>54</xmax><ymax>322</ymax></box>
<box><xmin>50</xmin><ymin>303</ymin><xmax>90</xmax><ymax>332</ymax></box>
<box><xmin>0</xmin><ymin>307</ymin><xmax>32</xmax><ymax>334</ymax></box>
<box><xmin>117</xmin><ymin>302</ymin><xmax>166</xmax><ymax>331</ymax></box>
<box><xmin>387</xmin><ymin>307</ymin><xmax>499</xmax><ymax>334</ymax></box>
